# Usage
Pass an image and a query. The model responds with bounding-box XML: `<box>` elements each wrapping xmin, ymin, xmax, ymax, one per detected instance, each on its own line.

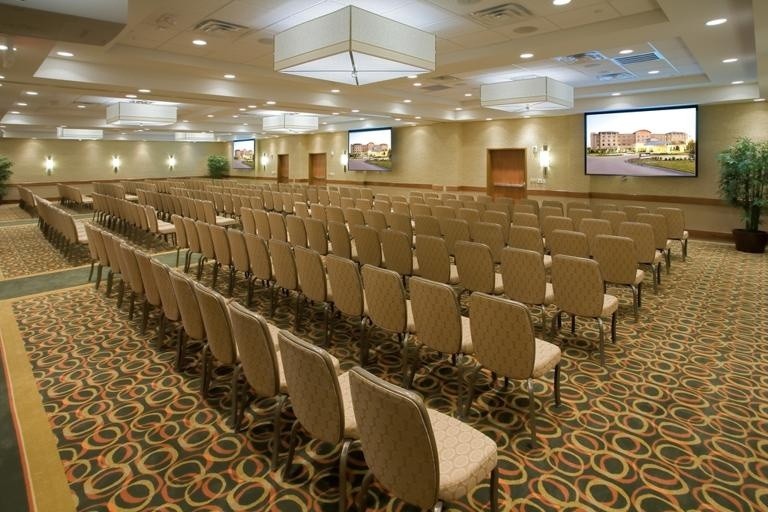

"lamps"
<box><xmin>263</xmin><ymin>114</ymin><xmax>319</xmax><ymax>135</ymax></box>
<box><xmin>111</xmin><ymin>154</ymin><xmax>120</xmax><ymax>174</ymax></box>
<box><xmin>175</xmin><ymin>132</ymin><xmax>215</xmax><ymax>144</ymax></box>
<box><xmin>340</xmin><ymin>150</ymin><xmax>348</xmax><ymax>172</ymax></box>
<box><xmin>261</xmin><ymin>153</ymin><xmax>267</xmax><ymax>170</ymax></box>
<box><xmin>539</xmin><ymin>145</ymin><xmax>551</xmax><ymax>177</ymax></box>
<box><xmin>272</xmin><ymin>5</ymin><xmax>436</xmax><ymax>86</ymax></box>
<box><xmin>106</xmin><ymin>102</ymin><xmax>177</xmax><ymax>128</ymax></box>
<box><xmin>167</xmin><ymin>153</ymin><xmax>176</xmax><ymax>172</ymax></box>
<box><xmin>480</xmin><ymin>76</ymin><xmax>574</xmax><ymax>115</ymax></box>
<box><xmin>42</xmin><ymin>153</ymin><xmax>55</xmax><ymax>176</ymax></box>
<box><xmin>56</xmin><ymin>127</ymin><xmax>103</xmax><ymax>140</ymax></box>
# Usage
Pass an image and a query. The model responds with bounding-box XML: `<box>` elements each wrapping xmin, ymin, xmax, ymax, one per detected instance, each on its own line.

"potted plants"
<box><xmin>718</xmin><ymin>138</ymin><xmax>768</xmax><ymax>253</ymax></box>
